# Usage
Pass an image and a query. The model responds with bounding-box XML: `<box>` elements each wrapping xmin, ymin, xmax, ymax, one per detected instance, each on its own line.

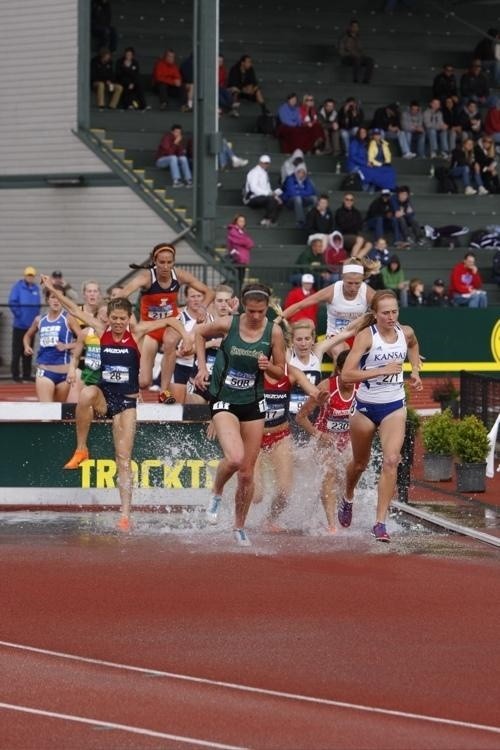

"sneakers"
<box><xmin>172</xmin><ymin>180</ymin><xmax>184</xmax><ymax>188</ymax></box>
<box><xmin>465</xmin><ymin>187</ymin><xmax>476</xmax><ymax>194</ymax></box>
<box><xmin>206</xmin><ymin>496</ymin><xmax>221</xmax><ymax>525</ymax></box>
<box><xmin>23</xmin><ymin>378</ymin><xmax>36</xmax><ymax>384</ymax></box>
<box><xmin>233</xmin><ymin>160</ymin><xmax>249</xmax><ymax>168</ymax></box>
<box><xmin>158</xmin><ymin>390</ymin><xmax>176</xmax><ymax>404</ymax></box>
<box><xmin>62</xmin><ymin>450</ymin><xmax>89</xmax><ymax>470</ymax></box>
<box><xmin>118</xmin><ymin>518</ymin><xmax>130</xmax><ymax>532</ymax></box>
<box><xmin>232</xmin><ymin>528</ymin><xmax>251</xmax><ymax>548</ymax></box>
<box><xmin>12</xmin><ymin>377</ymin><xmax>22</xmax><ymax>384</ymax></box>
<box><xmin>337</xmin><ymin>496</ymin><xmax>354</xmax><ymax>527</ymax></box>
<box><xmin>403</xmin><ymin>152</ymin><xmax>416</xmax><ymax>158</ymax></box>
<box><xmin>478</xmin><ymin>186</ymin><xmax>489</xmax><ymax>194</ymax></box>
<box><xmin>186</xmin><ymin>182</ymin><xmax>193</xmax><ymax>188</ymax></box>
<box><xmin>370</xmin><ymin>523</ymin><xmax>390</xmax><ymax>543</ymax></box>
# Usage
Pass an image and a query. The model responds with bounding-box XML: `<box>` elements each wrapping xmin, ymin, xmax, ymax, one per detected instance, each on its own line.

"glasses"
<box><xmin>345</xmin><ymin>198</ymin><xmax>354</xmax><ymax>201</ymax></box>
<box><xmin>306</xmin><ymin>99</ymin><xmax>313</xmax><ymax>101</ymax></box>
<box><xmin>474</xmin><ymin>65</ymin><xmax>481</xmax><ymax>67</ymax></box>
<box><xmin>483</xmin><ymin>139</ymin><xmax>492</xmax><ymax>143</ymax></box>
<box><xmin>447</xmin><ymin>69</ymin><xmax>453</xmax><ymax>71</ymax></box>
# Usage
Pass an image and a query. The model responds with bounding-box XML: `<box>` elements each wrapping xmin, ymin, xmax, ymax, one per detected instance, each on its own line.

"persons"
<box><xmin>460</xmin><ymin>59</ymin><xmax>500</xmax><ymax>110</ymax></box>
<box><xmin>8</xmin><ymin>267</ymin><xmax>41</xmax><ymax>385</ymax></box>
<box><xmin>219</xmin><ymin>137</ymin><xmax>249</xmax><ymax>171</ymax></box>
<box><xmin>365</xmin><ymin>189</ymin><xmax>411</xmax><ymax>248</ymax></box>
<box><xmin>369</xmin><ymin>105</ymin><xmax>417</xmax><ymax>159</ymax></box>
<box><xmin>299</xmin><ymin>96</ymin><xmax>325</xmax><ymax>155</ymax></box>
<box><xmin>286</xmin><ymin>318</ymin><xmax>355</xmax><ymax>448</ymax></box>
<box><xmin>218</xmin><ymin>59</ymin><xmax>241</xmax><ymax>117</ymax></box>
<box><xmin>346</xmin><ymin>128</ymin><xmax>370</xmax><ymax>190</ymax></box>
<box><xmin>274</xmin><ymin>257</ymin><xmax>376</xmax><ymax>377</ymax></box>
<box><xmin>301</xmin><ymin>194</ymin><xmax>334</xmax><ymax>244</ymax></box>
<box><xmin>228</xmin><ymin>55</ymin><xmax>269</xmax><ymax>117</ymax></box>
<box><xmin>484</xmin><ymin>99</ymin><xmax>500</xmax><ymax>154</ymax></box>
<box><xmin>368</xmin><ymin>130</ymin><xmax>397</xmax><ymax>193</ymax></box>
<box><xmin>23</xmin><ymin>284</ymin><xmax>81</xmax><ymax>402</ymax></box>
<box><xmin>368</xmin><ymin>237</ymin><xmax>390</xmax><ymax>265</ymax></box>
<box><xmin>295</xmin><ymin>350</ymin><xmax>363</xmax><ymax>534</ymax></box>
<box><xmin>427</xmin><ymin>279</ymin><xmax>449</xmax><ymax>306</ymax></box>
<box><xmin>252</xmin><ymin>335</ymin><xmax>331</xmax><ymax>533</ymax></box>
<box><xmin>66</xmin><ymin>305</ymin><xmax>110</xmax><ymax>387</ymax></box>
<box><xmin>441</xmin><ymin>99</ymin><xmax>469</xmax><ymax>160</ymax></box>
<box><xmin>320</xmin><ymin>99</ymin><xmax>345</xmax><ymax>158</ymax></box>
<box><xmin>182</xmin><ymin>49</ymin><xmax>196</xmax><ymax>109</ymax></box>
<box><xmin>337</xmin><ymin>20</ymin><xmax>374</xmax><ymax>84</ymax></box>
<box><xmin>180</xmin><ymin>285</ymin><xmax>240</xmax><ymax>404</ymax></box>
<box><xmin>117</xmin><ymin>243</ymin><xmax>215</xmax><ymax>404</ymax></box>
<box><xmin>174</xmin><ymin>285</ymin><xmax>215</xmax><ymax>403</ymax></box>
<box><xmin>106</xmin><ymin>286</ymin><xmax>136</xmax><ymax>330</ymax></box>
<box><xmin>194</xmin><ymin>285</ymin><xmax>285</xmax><ymax>547</ymax></box>
<box><xmin>339</xmin><ymin>99</ymin><xmax>361</xmax><ymax>154</ymax></box>
<box><xmin>423</xmin><ymin>98</ymin><xmax>448</xmax><ymax>157</ymax></box>
<box><xmin>226</xmin><ymin>215</ymin><xmax>255</xmax><ymax>288</ymax></box>
<box><xmin>473</xmin><ymin>136</ymin><xmax>500</xmax><ymax>195</ymax></box>
<box><xmin>433</xmin><ymin>64</ymin><xmax>459</xmax><ymax>106</ymax></box>
<box><xmin>448</xmin><ymin>254</ymin><xmax>488</xmax><ymax>309</ymax></box>
<box><xmin>449</xmin><ymin>138</ymin><xmax>489</xmax><ymax>195</ymax></box>
<box><xmin>282</xmin><ymin>161</ymin><xmax>318</xmax><ymax>228</ymax></box>
<box><xmin>462</xmin><ymin>102</ymin><xmax>485</xmax><ymax>140</ymax></box>
<box><xmin>241</xmin><ymin>153</ymin><xmax>283</xmax><ymax>229</ymax></box>
<box><xmin>42</xmin><ymin>271</ymin><xmax>79</xmax><ymax>300</ymax></box>
<box><xmin>155</xmin><ymin>125</ymin><xmax>193</xmax><ymax>189</ymax></box>
<box><xmin>283</xmin><ymin>273</ymin><xmax>319</xmax><ymax>328</ymax></box>
<box><xmin>90</xmin><ymin>49</ymin><xmax>124</xmax><ymax>111</ymax></box>
<box><xmin>287</xmin><ymin>239</ymin><xmax>331</xmax><ymax>292</ymax></box>
<box><xmin>76</xmin><ymin>281</ymin><xmax>101</xmax><ymax>329</ymax></box>
<box><xmin>390</xmin><ymin>185</ymin><xmax>424</xmax><ymax>246</ymax></box>
<box><xmin>471</xmin><ymin>30</ymin><xmax>500</xmax><ymax>90</ymax></box>
<box><xmin>90</xmin><ymin>0</ymin><xmax>118</xmax><ymax>54</ymax></box>
<box><xmin>401</xmin><ymin>102</ymin><xmax>427</xmax><ymax>160</ymax></box>
<box><xmin>280</xmin><ymin>149</ymin><xmax>312</xmax><ymax>185</ymax></box>
<box><xmin>324</xmin><ymin>231</ymin><xmax>348</xmax><ymax>282</ymax></box>
<box><xmin>338</xmin><ymin>290</ymin><xmax>423</xmax><ymax>542</ymax></box>
<box><xmin>407</xmin><ymin>279</ymin><xmax>429</xmax><ymax>305</ymax></box>
<box><xmin>335</xmin><ymin>193</ymin><xmax>373</xmax><ymax>259</ymax></box>
<box><xmin>40</xmin><ymin>275</ymin><xmax>192</xmax><ymax>526</ymax></box>
<box><xmin>116</xmin><ymin>46</ymin><xmax>151</xmax><ymax>111</ymax></box>
<box><xmin>380</xmin><ymin>255</ymin><xmax>408</xmax><ymax>305</ymax></box>
<box><xmin>153</xmin><ymin>50</ymin><xmax>187</xmax><ymax>112</ymax></box>
<box><xmin>277</xmin><ymin>96</ymin><xmax>313</xmax><ymax>157</ymax></box>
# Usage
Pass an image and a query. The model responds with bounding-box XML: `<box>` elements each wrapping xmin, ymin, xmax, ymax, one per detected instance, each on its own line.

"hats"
<box><xmin>301</xmin><ymin>274</ymin><xmax>314</xmax><ymax>284</ymax></box>
<box><xmin>51</xmin><ymin>271</ymin><xmax>63</xmax><ymax>279</ymax></box>
<box><xmin>435</xmin><ymin>280</ymin><xmax>445</xmax><ymax>287</ymax></box>
<box><xmin>382</xmin><ymin>189</ymin><xmax>390</xmax><ymax>195</ymax></box>
<box><xmin>24</xmin><ymin>267</ymin><xmax>36</xmax><ymax>276</ymax></box>
<box><xmin>372</xmin><ymin>128</ymin><xmax>380</xmax><ymax>133</ymax></box>
<box><xmin>259</xmin><ymin>155</ymin><xmax>271</xmax><ymax>164</ymax></box>
